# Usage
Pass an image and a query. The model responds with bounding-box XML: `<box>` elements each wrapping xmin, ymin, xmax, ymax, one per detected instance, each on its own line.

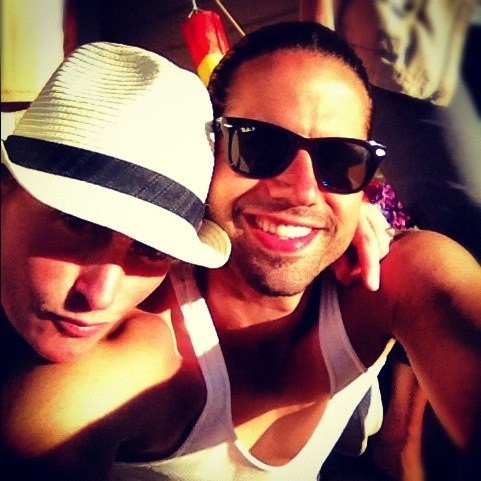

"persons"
<box><xmin>298</xmin><ymin>0</ymin><xmax>479</xmax><ymax>479</ymax></box>
<box><xmin>2</xmin><ymin>18</ymin><xmax>481</xmax><ymax>481</ymax></box>
<box><xmin>0</xmin><ymin>38</ymin><xmax>216</xmax><ymax>367</ymax></box>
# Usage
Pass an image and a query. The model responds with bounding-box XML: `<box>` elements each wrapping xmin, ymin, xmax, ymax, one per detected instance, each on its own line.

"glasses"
<box><xmin>207</xmin><ymin>113</ymin><xmax>387</xmax><ymax>198</ymax></box>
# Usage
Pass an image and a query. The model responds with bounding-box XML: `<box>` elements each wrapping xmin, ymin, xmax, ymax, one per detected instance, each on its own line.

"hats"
<box><xmin>0</xmin><ymin>39</ymin><xmax>231</xmax><ymax>268</ymax></box>
